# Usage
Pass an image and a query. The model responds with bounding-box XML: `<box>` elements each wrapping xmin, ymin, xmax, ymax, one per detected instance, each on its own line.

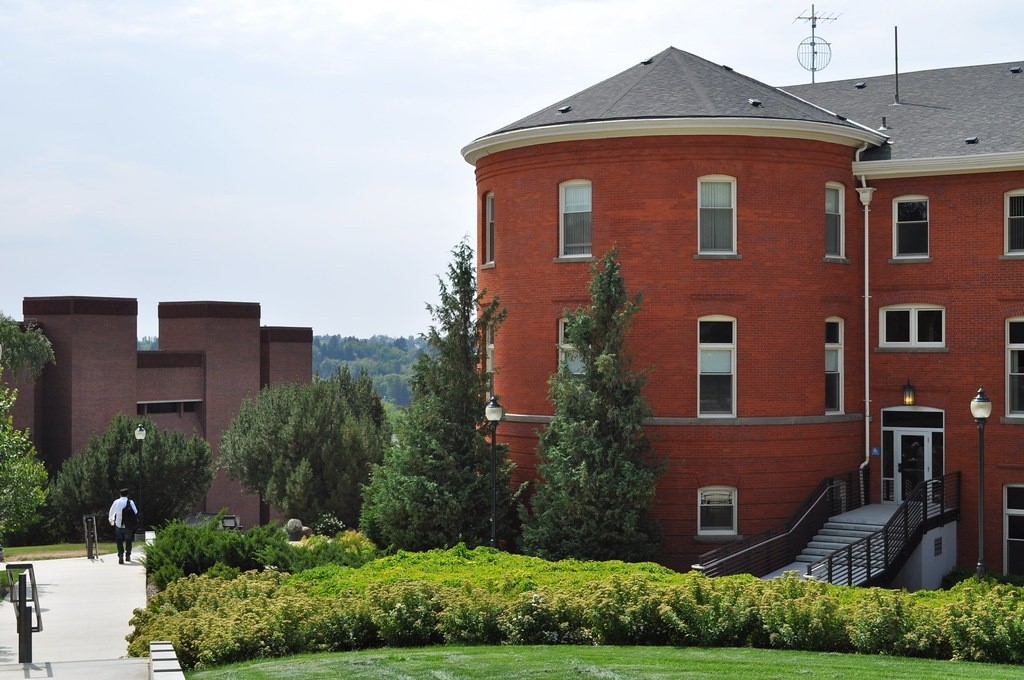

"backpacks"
<box><xmin>121</xmin><ymin>498</ymin><xmax>137</xmax><ymax>530</ymax></box>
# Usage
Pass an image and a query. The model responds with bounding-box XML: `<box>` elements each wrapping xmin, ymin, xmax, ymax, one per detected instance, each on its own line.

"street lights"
<box><xmin>134</xmin><ymin>424</ymin><xmax>146</xmax><ymax>540</ymax></box>
<box><xmin>485</xmin><ymin>396</ymin><xmax>502</xmax><ymax>547</ymax></box>
<box><xmin>970</xmin><ymin>388</ymin><xmax>992</xmax><ymax>578</ymax></box>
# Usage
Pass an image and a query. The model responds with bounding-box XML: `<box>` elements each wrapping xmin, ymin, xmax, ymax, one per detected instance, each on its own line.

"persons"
<box><xmin>109</xmin><ymin>488</ymin><xmax>138</xmax><ymax>564</ymax></box>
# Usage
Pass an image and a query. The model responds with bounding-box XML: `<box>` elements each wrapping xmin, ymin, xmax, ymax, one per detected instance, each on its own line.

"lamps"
<box><xmin>902</xmin><ymin>378</ymin><xmax>915</xmax><ymax>405</ymax></box>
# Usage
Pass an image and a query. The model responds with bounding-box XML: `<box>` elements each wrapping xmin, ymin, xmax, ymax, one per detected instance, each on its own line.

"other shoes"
<box><xmin>126</xmin><ymin>554</ymin><xmax>130</xmax><ymax>561</ymax></box>
<box><xmin>118</xmin><ymin>553</ymin><xmax>123</xmax><ymax>564</ymax></box>
